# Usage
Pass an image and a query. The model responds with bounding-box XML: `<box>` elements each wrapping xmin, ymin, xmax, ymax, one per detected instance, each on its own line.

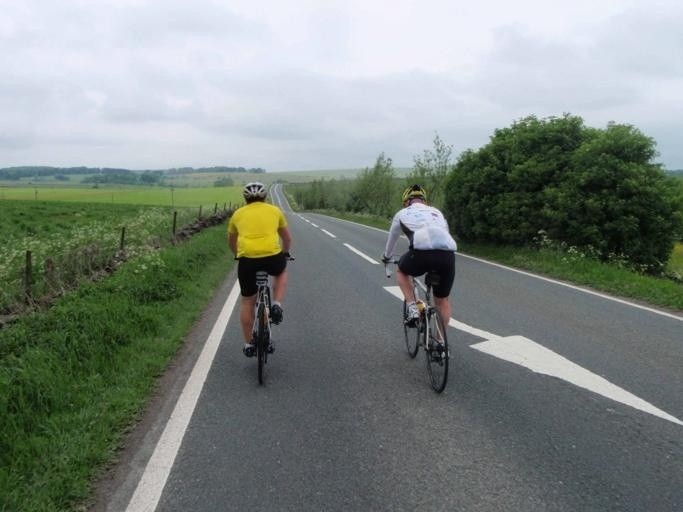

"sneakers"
<box><xmin>403</xmin><ymin>303</ymin><xmax>421</xmax><ymax>326</ymax></box>
<box><xmin>431</xmin><ymin>338</ymin><xmax>450</xmax><ymax>363</ymax></box>
<box><xmin>269</xmin><ymin>304</ymin><xmax>284</xmax><ymax>326</ymax></box>
<box><xmin>242</xmin><ymin>342</ymin><xmax>257</xmax><ymax>360</ymax></box>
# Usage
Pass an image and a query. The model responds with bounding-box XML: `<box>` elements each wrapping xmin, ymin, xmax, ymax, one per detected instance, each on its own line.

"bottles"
<box><xmin>417</xmin><ymin>299</ymin><xmax>425</xmax><ymax>312</ymax></box>
<box><xmin>386</xmin><ymin>256</ymin><xmax>394</xmax><ymax>278</ymax></box>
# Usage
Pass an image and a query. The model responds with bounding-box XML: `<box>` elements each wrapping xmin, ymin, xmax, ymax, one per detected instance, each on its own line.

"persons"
<box><xmin>225</xmin><ymin>182</ymin><xmax>291</xmax><ymax>357</ymax></box>
<box><xmin>380</xmin><ymin>183</ymin><xmax>457</xmax><ymax>357</ymax></box>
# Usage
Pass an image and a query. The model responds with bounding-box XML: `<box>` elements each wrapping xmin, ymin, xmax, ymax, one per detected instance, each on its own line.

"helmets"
<box><xmin>242</xmin><ymin>182</ymin><xmax>267</xmax><ymax>200</ymax></box>
<box><xmin>402</xmin><ymin>183</ymin><xmax>427</xmax><ymax>202</ymax></box>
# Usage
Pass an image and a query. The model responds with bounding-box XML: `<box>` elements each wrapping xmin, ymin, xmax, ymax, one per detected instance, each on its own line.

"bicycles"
<box><xmin>234</xmin><ymin>256</ymin><xmax>294</xmax><ymax>384</ymax></box>
<box><xmin>384</xmin><ymin>258</ymin><xmax>455</xmax><ymax>393</ymax></box>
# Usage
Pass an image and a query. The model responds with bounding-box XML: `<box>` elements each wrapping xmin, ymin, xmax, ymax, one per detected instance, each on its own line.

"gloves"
<box><xmin>381</xmin><ymin>251</ymin><xmax>394</xmax><ymax>265</ymax></box>
<box><xmin>281</xmin><ymin>249</ymin><xmax>292</xmax><ymax>261</ymax></box>
